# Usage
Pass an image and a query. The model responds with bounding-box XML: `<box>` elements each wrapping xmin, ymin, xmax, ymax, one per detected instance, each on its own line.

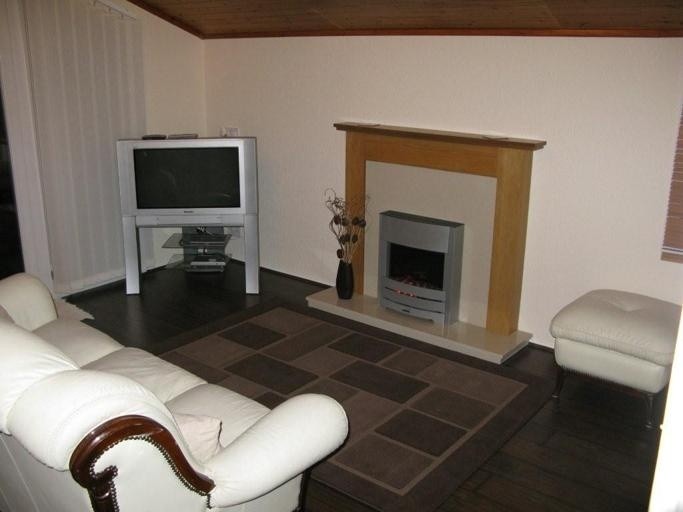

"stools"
<box><xmin>550</xmin><ymin>289</ymin><xmax>683</xmax><ymax>430</ymax></box>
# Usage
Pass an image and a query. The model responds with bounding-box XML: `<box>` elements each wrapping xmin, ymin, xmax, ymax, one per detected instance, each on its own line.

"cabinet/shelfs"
<box><xmin>122</xmin><ymin>214</ymin><xmax>261</xmax><ymax>295</ymax></box>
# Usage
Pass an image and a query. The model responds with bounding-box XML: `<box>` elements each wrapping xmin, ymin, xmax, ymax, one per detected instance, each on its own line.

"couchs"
<box><xmin>1</xmin><ymin>274</ymin><xmax>349</xmax><ymax>512</ymax></box>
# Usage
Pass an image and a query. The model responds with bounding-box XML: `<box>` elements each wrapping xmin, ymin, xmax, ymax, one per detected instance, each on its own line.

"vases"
<box><xmin>335</xmin><ymin>260</ymin><xmax>354</xmax><ymax>299</ymax></box>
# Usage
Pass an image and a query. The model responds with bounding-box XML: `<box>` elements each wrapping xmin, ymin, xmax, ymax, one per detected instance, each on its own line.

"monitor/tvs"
<box><xmin>115</xmin><ymin>138</ymin><xmax>257</xmax><ymax>227</ymax></box>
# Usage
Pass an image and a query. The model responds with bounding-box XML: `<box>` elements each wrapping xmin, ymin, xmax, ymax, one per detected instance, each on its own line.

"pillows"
<box><xmin>174</xmin><ymin>413</ymin><xmax>225</xmax><ymax>464</ymax></box>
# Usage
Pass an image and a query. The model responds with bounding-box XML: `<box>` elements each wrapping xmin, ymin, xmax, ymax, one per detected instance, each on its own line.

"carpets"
<box><xmin>157</xmin><ymin>307</ymin><xmax>563</xmax><ymax>511</ymax></box>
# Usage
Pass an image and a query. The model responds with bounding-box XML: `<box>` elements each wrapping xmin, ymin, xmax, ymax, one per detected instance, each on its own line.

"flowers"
<box><xmin>322</xmin><ymin>187</ymin><xmax>372</xmax><ymax>264</ymax></box>
